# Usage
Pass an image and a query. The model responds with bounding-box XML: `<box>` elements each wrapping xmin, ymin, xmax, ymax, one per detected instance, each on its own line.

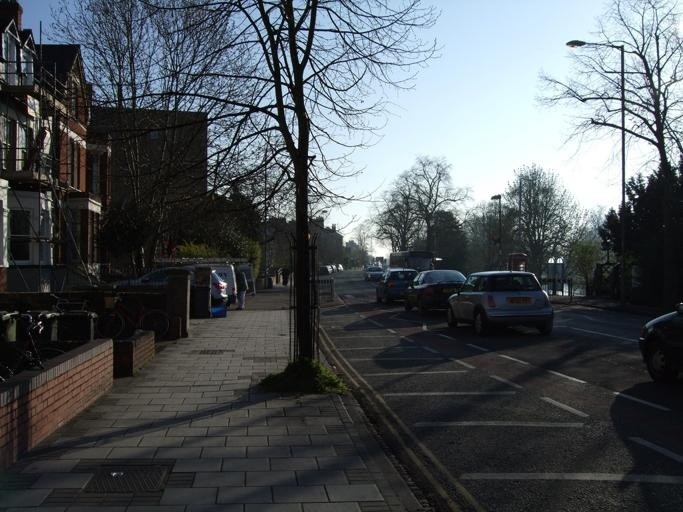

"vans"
<box><xmin>8</xmin><ymin>252</ymin><xmax>255</xmax><ymax>317</ymax></box>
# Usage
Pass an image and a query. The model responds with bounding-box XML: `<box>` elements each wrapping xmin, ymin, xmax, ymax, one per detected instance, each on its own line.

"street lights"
<box><xmin>565</xmin><ymin>40</ymin><xmax>627</xmax><ymax>303</ymax></box>
<box><xmin>547</xmin><ymin>255</ymin><xmax>556</xmax><ymax>295</ymax></box>
<box><xmin>556</xmin><ymin>256</ymin><xmax>565</xmax><ymax>297</ymax></box>
<box><xmin>490</xmin><ymin>194</ymin><xmax>503</xmax><ymax>271</ymax></box>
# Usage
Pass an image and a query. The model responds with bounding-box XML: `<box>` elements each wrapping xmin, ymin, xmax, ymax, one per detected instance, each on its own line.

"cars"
<box><xmin>321</xmin><ymin>261</ymin><xmax>344</xmax><ymax>277</ymax></box>
<box><xmin>367</xmin><ymin>251</ymin><xmax>554</xmax><ymax>339</ymax></box>
<box><xmin>637</xmin><ymin>302</ymin><xmax>682</xmax><ymax>401</ymax></box>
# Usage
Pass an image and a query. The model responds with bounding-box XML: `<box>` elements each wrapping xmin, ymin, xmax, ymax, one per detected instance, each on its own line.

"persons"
<box><xmin>235</xmin><ymin>270</ymin><xmax>248</xmax><ymax>310</ymax></box>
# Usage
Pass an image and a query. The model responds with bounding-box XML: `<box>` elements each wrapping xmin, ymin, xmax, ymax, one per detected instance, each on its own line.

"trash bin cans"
<box><xmin>268</xmin><ymin>274</ymin><xmax>276</xmax><ymax>289</ymax></box>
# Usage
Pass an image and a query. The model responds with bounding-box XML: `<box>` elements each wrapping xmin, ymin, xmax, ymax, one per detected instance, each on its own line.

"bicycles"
<box><xmin>1</xmin><ymin>304</ymin><xmax>67</xmax><ymax>384</ymax></box>
